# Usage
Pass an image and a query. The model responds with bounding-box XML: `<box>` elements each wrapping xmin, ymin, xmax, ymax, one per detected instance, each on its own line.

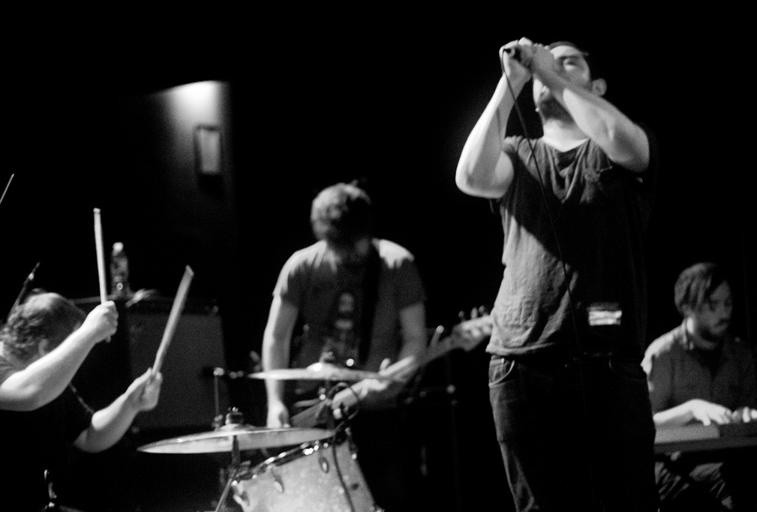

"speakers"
<box><xmin>73</xmin><ymin>292</ymin><xmax>225</xmax><ymax>436</ymax></box>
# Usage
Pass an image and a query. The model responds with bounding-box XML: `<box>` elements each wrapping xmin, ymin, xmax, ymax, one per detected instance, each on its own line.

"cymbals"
<box><xmin>250</xmin><ymin>364</ymin><xmax>387</xmax><ymax>381</ymax></box>
<box><xmin>139</xmin><ymin>424</ymin><xmax>335</xmax><ymax>454</ymax></box>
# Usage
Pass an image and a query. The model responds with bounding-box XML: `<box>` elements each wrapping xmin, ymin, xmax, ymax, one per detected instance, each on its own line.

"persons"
<box><xmin>640</xmin><ymin>258</ymin><xmax>757</xmax><ymax>512</ymax></box>
<box><xmin>259</xmin><ymin>181</ymin><xmax>429</xmax><ymax>434</ymax></box>
<box><xmin>452</xmin><ymin>32</ymin><xmax>663</xmax><ymax>510</ymax></box>
<box><xmin>0</xmin><ymin>290</ymin><xmax>164</xmax><ymax>512</ymax></box>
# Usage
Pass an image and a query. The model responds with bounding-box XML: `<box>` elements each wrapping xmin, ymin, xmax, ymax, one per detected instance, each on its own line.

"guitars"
<box><xmin>263</xmin><ymin>305</ymin><xmax>492</xmax><ymax>458</ymax></box>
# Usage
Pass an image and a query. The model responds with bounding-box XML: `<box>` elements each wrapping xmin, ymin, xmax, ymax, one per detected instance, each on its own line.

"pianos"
<box><xmin>654</xmin><ymin>423</ymin><xmax>757</xmax><ymax>453</ymax></box>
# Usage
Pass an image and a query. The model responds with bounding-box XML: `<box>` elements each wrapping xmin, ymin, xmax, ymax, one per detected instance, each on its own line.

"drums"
<box><xmin>231</xmin><ymin>431</ymin><xmax>384</xmax><ymax>512</ymax></box>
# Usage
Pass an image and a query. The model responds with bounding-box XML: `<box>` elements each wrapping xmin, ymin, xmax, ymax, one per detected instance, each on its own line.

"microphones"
<box><xmin>505</xmin><ymin>46</ymin><xmax>525</xmax><ymax>71</ymax></box>
<box><xmin>200</xmin><ymin>361</ymin><xmax>239</xmax><ymax>381</ymax></box>
